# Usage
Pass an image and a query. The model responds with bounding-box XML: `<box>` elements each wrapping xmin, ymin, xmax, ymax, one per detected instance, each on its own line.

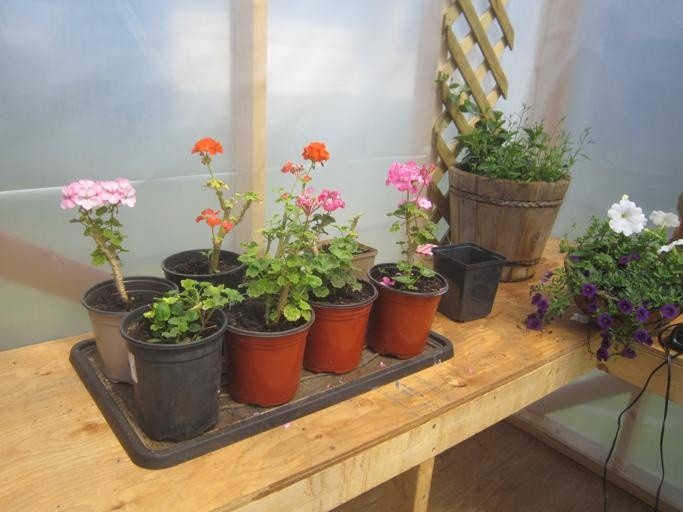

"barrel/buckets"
<box><xmin>447</xmin><ymin>164</ymin><xmax>572</xmax><ymax>284</ymax></box>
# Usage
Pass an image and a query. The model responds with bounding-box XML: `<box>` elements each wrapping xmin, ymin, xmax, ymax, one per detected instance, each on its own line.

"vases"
<box><xmin>560</xmin><ymin>255</ymin><xmax>683</xmax><ymax>340</ymax></box>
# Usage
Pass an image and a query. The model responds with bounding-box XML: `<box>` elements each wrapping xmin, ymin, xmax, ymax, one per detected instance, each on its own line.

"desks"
<box><xmin>0</xmin><ymin>237</ymin><xmax>683</xmax><ymax>510</ymax></box>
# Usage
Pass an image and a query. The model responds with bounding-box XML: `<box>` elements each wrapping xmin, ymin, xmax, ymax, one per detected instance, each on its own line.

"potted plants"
<box><xmin>436</xmin><ymin>71</ymin><xmax>595</xmax><ymax>281</ymax></box>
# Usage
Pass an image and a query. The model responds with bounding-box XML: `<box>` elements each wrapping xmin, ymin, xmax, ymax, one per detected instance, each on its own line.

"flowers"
<box><xmin>523</xmin><ymin>194</ymin><xmax>683</xmax><ymax>360</ymax></box>
